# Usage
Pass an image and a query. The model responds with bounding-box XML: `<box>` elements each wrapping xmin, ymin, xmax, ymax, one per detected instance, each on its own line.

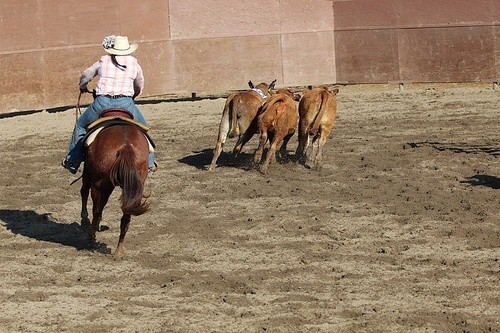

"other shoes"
<box><xmin>63</xmin><ymin>157</ymin><xmax>77</xmax><ymax>174</ymax></box>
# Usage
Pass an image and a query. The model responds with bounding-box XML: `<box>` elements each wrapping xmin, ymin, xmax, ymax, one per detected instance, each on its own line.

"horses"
<box><xmin>78</xmin><ymin>88</ymin><xmax>154</xmax><ymax>257</ymax></box>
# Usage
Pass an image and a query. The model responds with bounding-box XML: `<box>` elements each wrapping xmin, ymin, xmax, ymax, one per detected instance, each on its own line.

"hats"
<box><xmin>103</xmin><ymin>36</ymin><xmax>139</xmax><ymax>55</ymax></box>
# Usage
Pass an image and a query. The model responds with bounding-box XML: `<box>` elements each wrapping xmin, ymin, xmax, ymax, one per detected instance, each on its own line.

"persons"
<box><xmin>61</xmin><ymin>35</ymin><xmax>158</xmax><ymax>175</ymax></box>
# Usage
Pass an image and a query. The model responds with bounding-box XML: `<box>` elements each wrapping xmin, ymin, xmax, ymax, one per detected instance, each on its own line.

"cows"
<box><xmin>208</xmin><ymin>77</ymin><xmax>342</xmax><ymax>175</ymax></box>
